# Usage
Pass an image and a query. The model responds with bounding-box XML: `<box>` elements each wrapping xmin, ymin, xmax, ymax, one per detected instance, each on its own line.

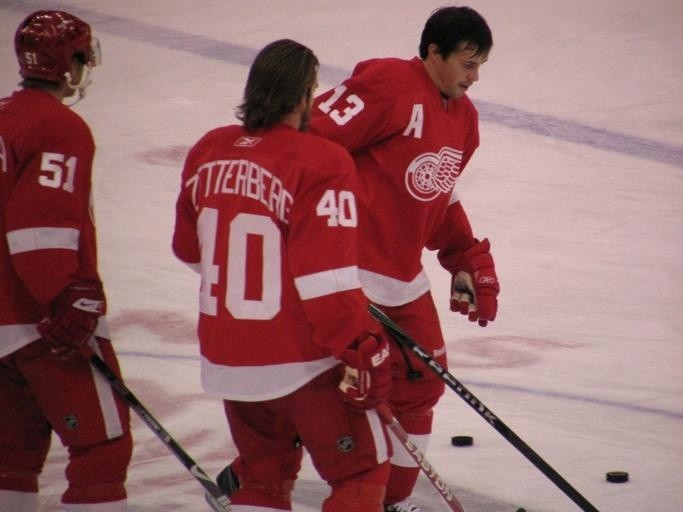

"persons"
<box><xmin>205</xmin><ymin>7</ymin><xmax>500</xmax><ymax>512</ymax></box>
<box><xmin>1</xmin><ymin>10</ymin><xmax>133</xmax><ymax>510</ymax></box>
<box><xmin>172</xmin><ymin>39</ymin><xmax>394</xmax><ymax>512</ymax></box>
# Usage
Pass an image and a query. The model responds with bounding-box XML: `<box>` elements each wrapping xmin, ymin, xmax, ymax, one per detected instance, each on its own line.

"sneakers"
<box><xmin>383</xmin><ymin>500</ymin><xmax>427</xmax><ymax>512</ymax></box>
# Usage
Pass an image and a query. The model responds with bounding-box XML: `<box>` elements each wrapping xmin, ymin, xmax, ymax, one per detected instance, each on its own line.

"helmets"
<box><xmin>12</xmin><ymin>9</ymin><xmax>102</xmax><ymax>108</ymax></box>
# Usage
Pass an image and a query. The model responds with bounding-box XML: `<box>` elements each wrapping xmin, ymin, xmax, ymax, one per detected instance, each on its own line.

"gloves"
<box><xmin>333</xmin><ymin>319</ymin><xmax>395</xmax><ymax>417</ymax></box>
<box><xmin>35</xmin><ymin>279</ymin><xmax>107</xmax><ymax>359</ymax></box>
<box><xmin>436</xmin><ymin>237</ymin><xmax>501</xmax><ymax>328</ymax></box>
